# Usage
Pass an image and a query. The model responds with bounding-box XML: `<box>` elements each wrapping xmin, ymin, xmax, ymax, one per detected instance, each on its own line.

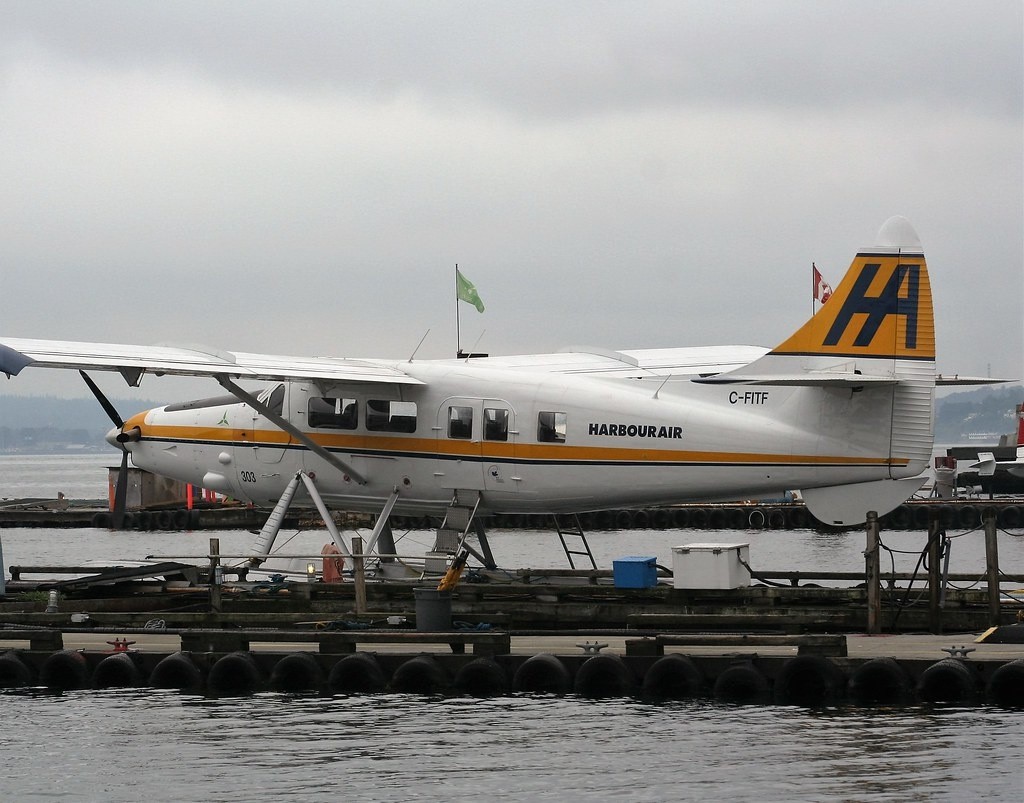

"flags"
<box><xmin>813</xmin><ymin>265</ymin><xmax>833</xmax><ymax>304</ymax></box>
<box><xmin>456</xmin><ymin>270</ymin><xmax>485</xmax><ymax>313</ymax></box>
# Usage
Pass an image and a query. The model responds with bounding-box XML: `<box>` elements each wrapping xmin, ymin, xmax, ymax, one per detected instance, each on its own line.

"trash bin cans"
<box><xmin>936</xmin><ymin>468</ymin><xmax>953</xmax><ymax>498</ymax></box>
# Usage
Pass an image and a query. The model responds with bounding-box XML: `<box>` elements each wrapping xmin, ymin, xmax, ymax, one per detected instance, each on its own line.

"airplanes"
<box><xmin>0</xmin><ymin>218</ymin><xmax>1020</xmax><ymax>579</ymax></box>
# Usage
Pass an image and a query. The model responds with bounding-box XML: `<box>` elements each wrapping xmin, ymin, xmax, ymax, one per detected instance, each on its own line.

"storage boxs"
<box><xmin>613</xmin><ymin>556</ymin><xmax>657</xmax><ymax>589</ymax></box>
<box><xmin>677</xmin><ymin>543</ymin><xmax>751</xmax><ymax>587</ymax></box>
